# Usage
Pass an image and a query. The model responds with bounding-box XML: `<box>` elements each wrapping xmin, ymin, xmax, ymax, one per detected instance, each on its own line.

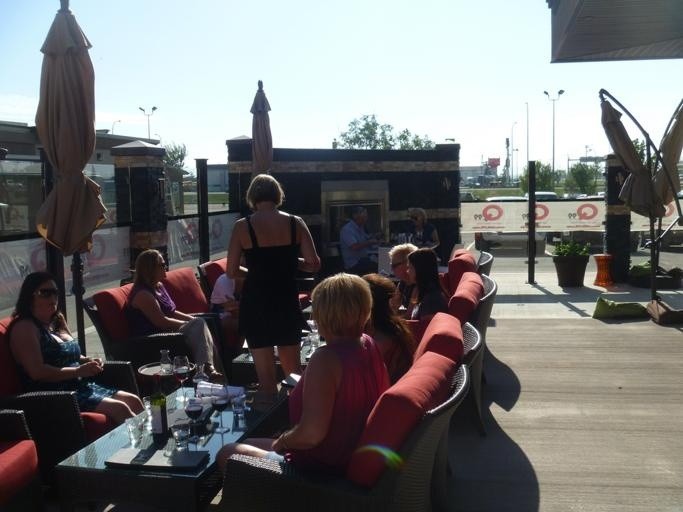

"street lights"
<box><xmin>137</xmin><ymin>105</ymin><xmax>158</xmax><ymax>139</ymax></box>
<box><xmin>542</xmin><ymin>89</ymin><xmax>564</xmax><ymax>173</ymax></box>
<box><xmin>585</xmin><ymin>144</ymin><xmax>592</xmax><ymax>167</ymax></box>
<box><xmin>511</xmin><ymin>121</ymin><xmax>519</xmax><ymax>183</ymax></box>
<box><xmin>524</xmin><ymin>102</ymin><xmax>529</xmax><ymax>161</ymax></box>
<box><xmin>153</xmin><ymin>133</ymin><xmax>162</xmax><ymax>148</ymax></box>
<box><xmin>111</xmin><ymin>120</ymin><xmax>120</xmax><ymax>134</ymax></box>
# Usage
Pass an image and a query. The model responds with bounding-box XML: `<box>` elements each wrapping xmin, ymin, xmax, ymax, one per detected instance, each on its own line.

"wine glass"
<box><xmin>158</xmin><ymin>349</ymin><xmax>192</xmax><ymax>405</ymax></box>
<box><xmin>183</xmin><ymin>381</ymin><xmax>231</xmax><ymax>439</ymax></box>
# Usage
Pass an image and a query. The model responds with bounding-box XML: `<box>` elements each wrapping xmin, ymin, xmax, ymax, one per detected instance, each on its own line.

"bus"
<box><xmin>1</xmin><ymin>147</ymin><xmax>189</xmax><ymax>310</ymax></box>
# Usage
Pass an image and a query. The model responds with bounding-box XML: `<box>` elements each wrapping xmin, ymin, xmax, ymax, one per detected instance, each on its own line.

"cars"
<box><xmin>458</xmin><ymin>189</ymin><xmax>683</xmax><ymax>256</ymax></box>
<box><xmin>461</xmin><ymin>176</ymin><xmax>519</xmax><ymax>187</ymax></box>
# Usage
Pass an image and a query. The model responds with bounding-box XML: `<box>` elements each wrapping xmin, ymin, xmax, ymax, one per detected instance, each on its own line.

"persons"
<box><xmin>120</xmin><ymin>250</ymin><xmax>229</xmax><ymax>390</ymax></box>
<box><xmin>2</xmin><ymin>271</ymin><xmax>144</xmax><ymax>426</ymax></box>
<box><xmin>359</xmin><ymin>270</ymin><xmax>416</xmax><ymax>388</ymax></box>
<box><xmin>225</xmin><ymin>174</ymin><xmax>323</xmax><ymax>389</ymax></box>
<box><xmin>337</xmin><ymin>205</ymin><xmax>385</xmax><ymax>277</ymax></box>
<box><xmin>208</xmin><ymin>272</ymin><xmax>236</xmax><ymax>315</ymax></box>
<box><xmin>405</xmin><ymin>246</ymin><xmax>449</xmax><ymax>322</ymax></box>
<box><xmin>393</xmin><ymin>206</ymin><xmax>440</xmax><ymax>253</ymax></box>
<box><xmin>386</xmin><ymin>241</ymin><xmax>419</xmax><ymax>309</ymax></box>
<box><xmin>214</xmin><ymin>268</ymin><xmax>394</xmax><ymax>488</ymax></box>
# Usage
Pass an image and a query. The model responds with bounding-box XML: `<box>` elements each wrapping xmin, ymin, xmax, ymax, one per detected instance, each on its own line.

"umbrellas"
<box><xmin>31</xmin><ymin>0</ymin><xmax>109</xmax><ymax>362</ymax></box>
<box><xmin>597</xmin><ymin>88</ymin><xmax>682</xmax><ymax>325</ymax></box>
<box><xmin>629</xmin><ymin>97</ymin><xmax>683</xmax><ymax>280</ymax></box>
<box><xmin>242</xmin><ymin>77</ymin><xmax>281</xmax><ymax>186</ymax></box>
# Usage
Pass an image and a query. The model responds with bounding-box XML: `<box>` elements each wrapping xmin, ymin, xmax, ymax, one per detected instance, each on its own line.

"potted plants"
<box><xmin>552</xmin><ymin>240</ymin><xmax>591</xmax><ymax>288</ymax></box>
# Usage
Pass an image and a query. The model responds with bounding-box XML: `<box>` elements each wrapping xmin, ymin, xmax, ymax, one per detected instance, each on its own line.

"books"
<box><xmin>102</xmin><ymin>444</ymin><xmax>210</xmax><ymax>471</ymax></box>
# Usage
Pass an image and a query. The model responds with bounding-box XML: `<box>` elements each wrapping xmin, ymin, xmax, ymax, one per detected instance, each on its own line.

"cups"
<box><xmin>124</xmin><ymin>417</ymin><xmax>146</xmax><ymax>442</ymax></box>
<box><xmin>142</xmin><ymin>395</ymin><xmax>149</xmax><ymax>413</ymax></box>
<box><xmin>394</xmin><ymin>232</ymin><xmax>427</xmax><ymax>245</ymax></box>
<box><xmin>300</xmin><ymin>315</ymin><xmax>321</xmax><ymax>352</ymax></box>
<box><xmin>169</xmin><ymin>424</ymin><xmax>193</xmax><ymax>452</ymax></box>
<box><xmin>232</xmin><ymin>393</ymin><xmax>248</xmax><ymax>417</ymax></box>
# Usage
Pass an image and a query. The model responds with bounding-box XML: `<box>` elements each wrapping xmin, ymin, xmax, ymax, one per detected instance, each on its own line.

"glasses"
<box><xmin>34</xmin><ymin>288</ymin><xmax>60</xmax><ymax>298</ymax></box>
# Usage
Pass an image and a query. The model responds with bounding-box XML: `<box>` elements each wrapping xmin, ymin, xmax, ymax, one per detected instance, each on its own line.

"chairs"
<box><xmin>0</xmin><ymin>315</ymin><xmax>144</xmax><ymax>450</ymax></box>
<box><xmin>225</xmin><ymin>364</ymin><xmax>469</xmax><ymax>510</ymax></box>
<box><xmin>81</xmin><ymin>259</ymin><xmax>238</xmax><ymax>387</ymax></box>
<box><xmin>199</xmin><ymin>258</ymin><xmax>310</xmax><ymax>310</ymax></box>
<box><xmin>1</xmin><ymin>410</ymin><xmax>54</xmax><ymax>511</ymax></box>
<box><xmin>410</xmin><ymin>248</ymin><xmax>497</xmax><ymax>437</ymax></box>
<box><xmin>378</xmin><ymin>248</ymin><xmax>394</xmax><ymax>277</ymax></box>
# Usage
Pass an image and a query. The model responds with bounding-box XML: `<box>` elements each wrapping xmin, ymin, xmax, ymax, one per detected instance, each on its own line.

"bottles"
<box><xmin>149</xmin><ymin>371</ymin><xmax>169</xmax><ymax>447</ymax></box>
